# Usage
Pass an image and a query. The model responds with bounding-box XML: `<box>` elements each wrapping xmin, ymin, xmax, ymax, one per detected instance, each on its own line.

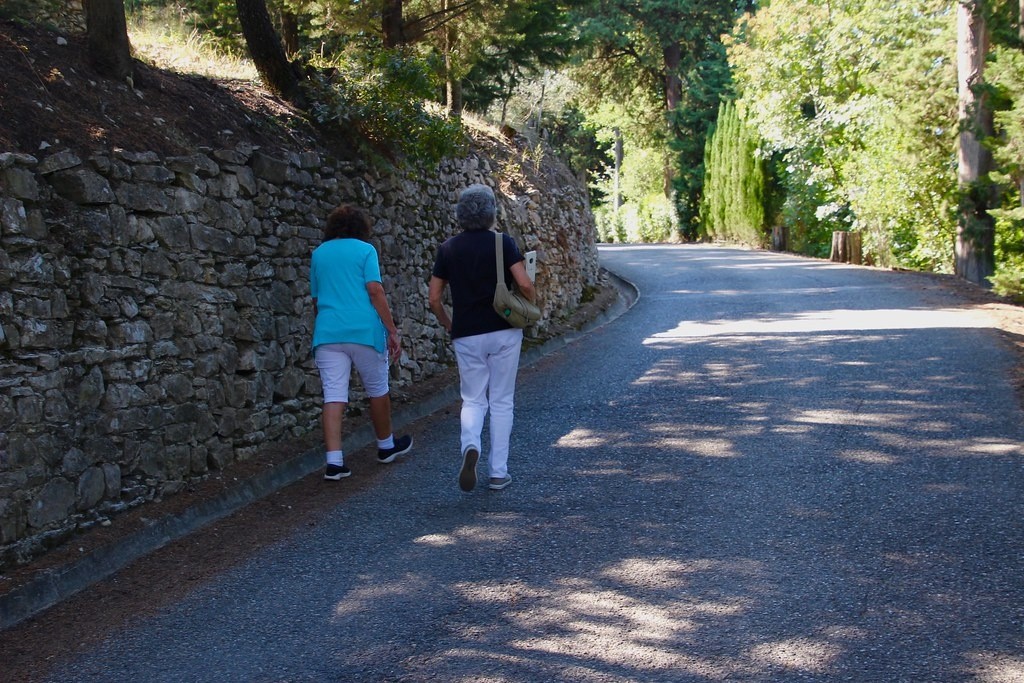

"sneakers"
<box><xmin>377</xmin><ymin>434</ymin><xmax>413</xmax><ymax>463</ymax></box>
<box><xmin>324</xmin><ymin>463</ymin><xmax>351</xmax><ymax>480</ymax></box>
<box><xmin>458</xmin><ymin>444</ymin><xmax>479</xmax><ymax>491</ymax></box>
<box><xmin>489</xmin><ymin>474</ymin><xmax>512</xmax><ymax>489</ymax></box>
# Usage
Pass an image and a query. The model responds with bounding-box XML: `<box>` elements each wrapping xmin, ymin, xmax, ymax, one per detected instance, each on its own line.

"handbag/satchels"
<box><xmin>493</xmin><ymin>233</ymin><xmax>541</xmax><ymax>328</ymax></box>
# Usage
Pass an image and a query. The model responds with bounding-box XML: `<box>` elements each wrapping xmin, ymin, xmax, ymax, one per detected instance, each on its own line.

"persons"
<box><xmin>310</xmin><ymin>204</ymin><xmax>413</xmax><ymax>480</ymax></box>
<box><xmin>428</xmin><ymin>183</ymin><xmax>536</xmax><ymax>491</ymax></box>
